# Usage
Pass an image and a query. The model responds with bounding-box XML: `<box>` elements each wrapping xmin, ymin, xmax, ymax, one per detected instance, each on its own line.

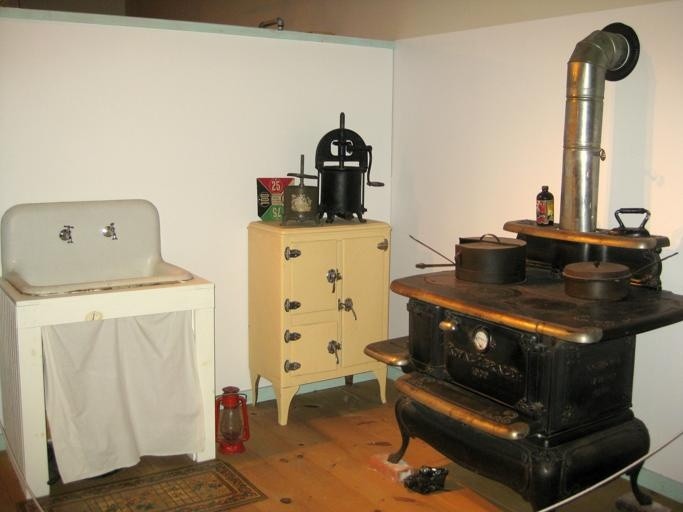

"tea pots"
<box><xmin>608</xmin><ymin>208</ymin><xmax>662</xmax><ymax>281</ymax></box>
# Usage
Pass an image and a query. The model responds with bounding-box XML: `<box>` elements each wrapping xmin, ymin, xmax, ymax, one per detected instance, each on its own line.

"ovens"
<box><xmin>387</xmin><ymin>269</ymin><xmax>683</xmax><ymax>511</ymax></box>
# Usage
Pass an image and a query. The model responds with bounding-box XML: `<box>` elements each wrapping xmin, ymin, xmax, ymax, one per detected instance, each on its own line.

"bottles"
<box><xmin>535</xmin><ymin>186</ymin><xmax>554</xmax><ymax>226</ymax></box>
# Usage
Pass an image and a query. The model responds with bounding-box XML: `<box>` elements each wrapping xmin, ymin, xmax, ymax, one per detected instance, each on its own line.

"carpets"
<box><xmin>17</xmin><ymin>458</ymin><xmax>268</xmax><ymax>512</ymax></box>
<box><xmin>438</xmin><ymin>462</ymin><xmax>682</xmax><ymax>512</ymax></box>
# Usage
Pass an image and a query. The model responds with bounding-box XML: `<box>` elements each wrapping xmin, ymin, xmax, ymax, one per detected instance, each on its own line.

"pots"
<box><xmin>561</xmin><ymin>252</ymin><xmax>678</xmax><ymax>300</ymax></box>
<box><xmin>408</xmin><ymin>233</ymin><xmax>525</xmax><ymax>286</ymax></box>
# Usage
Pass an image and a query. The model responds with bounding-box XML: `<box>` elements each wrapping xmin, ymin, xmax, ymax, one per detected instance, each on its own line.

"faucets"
<box><xmin>104</xmin><ymin>221</ymin><xmax>118</xmax><ymax>240</ymax></box>
<box><xmin>60</xmin><ymin>224</ymin><xmax>76</xmax><ymax>244</ymax></box>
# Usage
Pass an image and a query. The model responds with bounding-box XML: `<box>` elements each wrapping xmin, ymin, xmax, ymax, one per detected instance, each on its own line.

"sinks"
<box><xmin>1</xmin><ymin>200</ymin><xmax>195</xmax><ymax>294</ymax></box>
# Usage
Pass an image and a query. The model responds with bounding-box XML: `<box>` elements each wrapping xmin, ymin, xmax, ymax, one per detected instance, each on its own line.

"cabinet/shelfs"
<box><xmin>247</xmin><ymin>218</ymin><xmax>392</xmax><ymax>427</ymax></box>
